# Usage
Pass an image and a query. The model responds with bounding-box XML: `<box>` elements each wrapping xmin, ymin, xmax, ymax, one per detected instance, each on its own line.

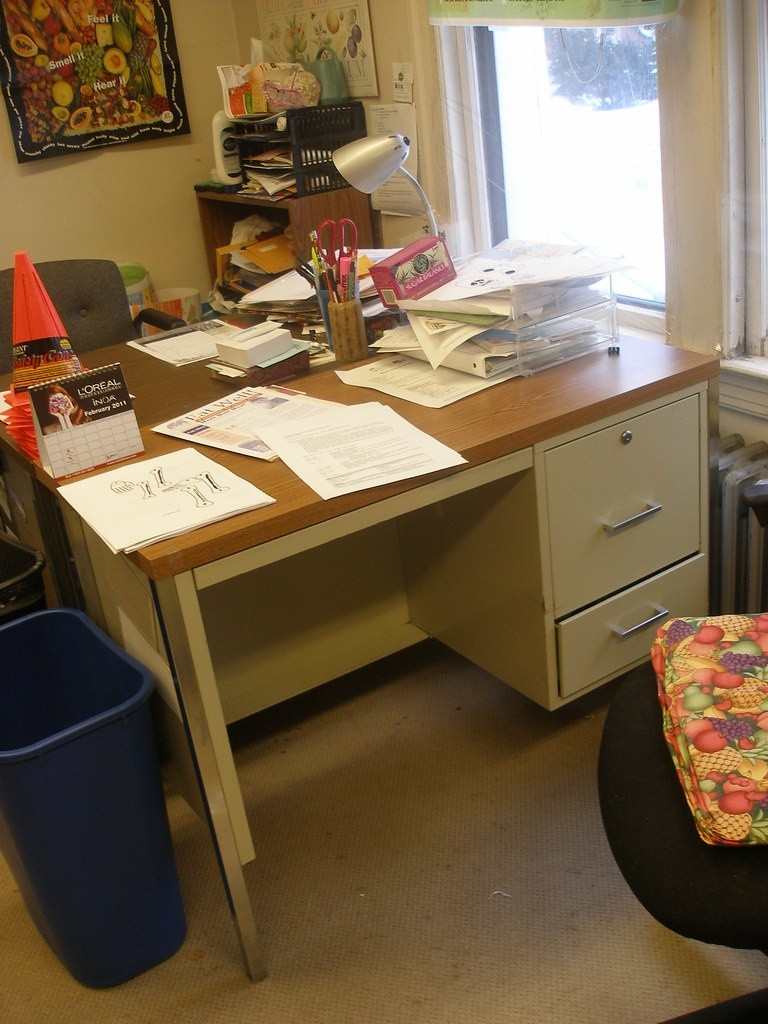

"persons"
<box><xmin>43</xmin><ymin>386</ymin><xmax>92</xmax><ymax>434</ymax></box>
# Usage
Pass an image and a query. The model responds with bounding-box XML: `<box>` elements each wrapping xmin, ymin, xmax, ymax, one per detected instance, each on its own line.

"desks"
<box><xmin>0</xmin><ymin>303</ymin><xmax>720</xmax><ymax>984</ymax></box>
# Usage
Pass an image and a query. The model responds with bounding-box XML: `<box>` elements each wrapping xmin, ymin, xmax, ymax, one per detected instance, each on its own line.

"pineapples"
<box><xmin>133</xmin><ymin>30</ymin><xmax>149</xmax><ymax>58</ymax></box>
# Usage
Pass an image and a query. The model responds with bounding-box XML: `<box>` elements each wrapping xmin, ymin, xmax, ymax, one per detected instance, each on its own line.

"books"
<box><xmin>471</xmin><ymin>329</ymin><xmax>549</xmax><ymax>354</ymax></box>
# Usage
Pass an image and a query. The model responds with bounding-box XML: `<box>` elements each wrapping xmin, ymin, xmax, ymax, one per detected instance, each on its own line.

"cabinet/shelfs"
<box><xmin>235</xmin><ymin>101</ymin><xmax>368</xmax><ymax>199</ymax></box>
<box><xmin>191</xmin><ymin>184</ymin><xmax>374</xmax><ymax>297</ymax></box>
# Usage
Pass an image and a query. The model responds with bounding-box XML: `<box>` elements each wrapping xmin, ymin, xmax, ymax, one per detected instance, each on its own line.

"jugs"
<box><xmin>294</xmin><ymin>45</ymin><xmax>348</xmax><ymax>106</ymax></box>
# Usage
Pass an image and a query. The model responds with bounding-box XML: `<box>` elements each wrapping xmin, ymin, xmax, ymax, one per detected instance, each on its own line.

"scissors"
<box><xmin>317</xmin><ymin>218</ymin><xmax>358</xmax><ymax>281</ymax></box>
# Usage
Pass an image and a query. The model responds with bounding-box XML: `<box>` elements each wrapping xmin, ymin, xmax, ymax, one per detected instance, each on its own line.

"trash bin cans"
<box><xmin>0</xmin><ymin>529</ymin><xmax>46</xmax><ymax>626</ymax></box>
<box><xmin>0</xmin><ymin>607</ymin><xmax>186</xmax><ymax>990</ymax></box>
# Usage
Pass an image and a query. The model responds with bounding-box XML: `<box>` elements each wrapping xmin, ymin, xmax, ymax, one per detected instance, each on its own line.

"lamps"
<box><xmin>330</xmin><ymin>133</ymin><xmax>438</xmax><ymax>237</ymax></box>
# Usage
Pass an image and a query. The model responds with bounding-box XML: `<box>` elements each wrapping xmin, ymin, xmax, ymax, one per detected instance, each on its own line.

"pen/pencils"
<box><xmin>295</xmin><ymin>230</ymin><xmax>359</xmax><ymax>303</ymax></box>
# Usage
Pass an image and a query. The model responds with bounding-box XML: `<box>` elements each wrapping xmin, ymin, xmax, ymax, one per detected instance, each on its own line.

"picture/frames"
<box><xmin>0</xmin><ymin>0</ymin><xmax>192</xmax><ymax>166</ymax></box>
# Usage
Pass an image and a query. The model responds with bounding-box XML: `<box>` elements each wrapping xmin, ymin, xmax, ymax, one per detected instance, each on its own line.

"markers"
<box><xmin>339</xmin><ymin>257</ymin><xmax>351</xmax><ymax>302</ymax></box>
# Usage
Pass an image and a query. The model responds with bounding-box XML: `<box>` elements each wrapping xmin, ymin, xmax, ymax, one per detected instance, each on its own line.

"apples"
<box><xmin>96</xmin><ymin>0</ymin><xmax>114</xmax><ymax>19</ymax></box>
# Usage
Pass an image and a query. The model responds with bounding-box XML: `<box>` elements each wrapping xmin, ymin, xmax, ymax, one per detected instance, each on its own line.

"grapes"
<box><xmin>71</xmin><ymin>43</ymin><xmax>107</xmax><ymax>83</ymax></box>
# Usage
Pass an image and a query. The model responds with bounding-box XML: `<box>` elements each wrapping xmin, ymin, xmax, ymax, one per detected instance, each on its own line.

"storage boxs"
<box><xmin>215</xmin><ymin>328</ymin><xmax>294</xmax><ymax>365</ymax></box>
<box><xmin>368</xmin><ymin>236</ymin><xmax>455</xmax><ymax>306</ymax></box>
<box><xmin>208</xmin><ymin>350</ymin><xmax>310</xmax><ymax>390</ymax></box>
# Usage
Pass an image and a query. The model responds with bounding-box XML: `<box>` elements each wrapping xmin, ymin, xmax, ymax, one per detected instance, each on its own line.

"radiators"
<box><xmin>707</xmin><ymin>434</ymin><xmax>768</xmax><ymax>616</ymax></box>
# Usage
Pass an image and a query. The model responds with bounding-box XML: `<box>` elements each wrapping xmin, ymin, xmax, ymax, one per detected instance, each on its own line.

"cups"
<box><xmin>316</xmin><ymin>287</ymin><xmax>336</xmax><ymax>353</ymax></box>
<box><xmin>327</xmin><ymin>299</ymin><xmax>368</xmax><ymax>361</ymax></box>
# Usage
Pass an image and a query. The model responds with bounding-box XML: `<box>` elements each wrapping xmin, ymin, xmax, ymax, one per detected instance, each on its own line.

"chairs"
<box><xmin>0</xmin><ymin>257</ymin><xmax>187</xmax><ymax>368</ymax></box>
<box><xmin>591</xmin><ymin>659</ymin><xmax>768</xmax><ymax>1024</ymax></box>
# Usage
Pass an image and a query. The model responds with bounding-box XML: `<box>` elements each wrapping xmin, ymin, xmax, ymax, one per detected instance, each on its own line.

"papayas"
<box><xmin>11</xmin><ymin>14</ymin><xmax>132</xmax><ymax>130</ymax></box>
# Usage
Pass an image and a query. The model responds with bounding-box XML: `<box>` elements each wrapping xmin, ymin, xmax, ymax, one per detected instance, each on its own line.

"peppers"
<box><xmin>31</xmin><ymin>1</ymin><xmax>75</xmax><ymax>77</ymax></box>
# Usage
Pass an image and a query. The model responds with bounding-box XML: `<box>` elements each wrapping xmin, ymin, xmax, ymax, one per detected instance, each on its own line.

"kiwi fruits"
<box><xmin>137</xmin><ymin>95</ymin><xmax>156</xmax><ymax>117</ymax></box>
